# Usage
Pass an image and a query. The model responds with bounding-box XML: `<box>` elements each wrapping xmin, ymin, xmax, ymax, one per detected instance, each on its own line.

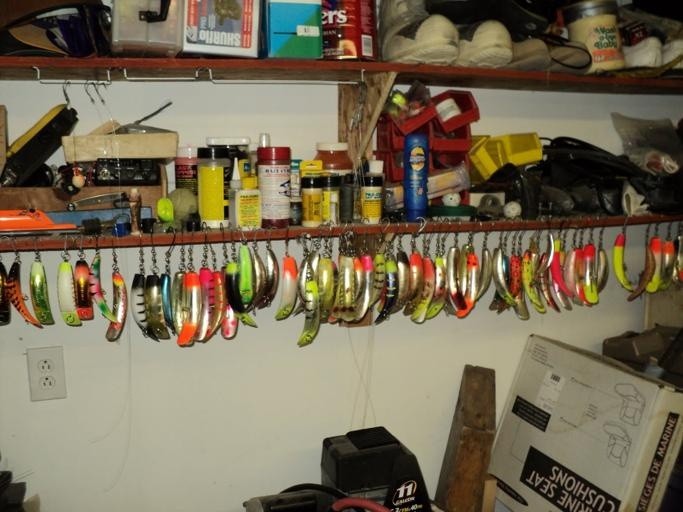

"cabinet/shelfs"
<box><xmin>0</xmin><ymin>54</ymin><xmax>681</xmax><ymax>253</ymax></box>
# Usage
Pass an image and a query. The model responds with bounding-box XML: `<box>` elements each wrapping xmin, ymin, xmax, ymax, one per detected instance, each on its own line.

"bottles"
<box><xmin>176</xmin><ymin>133</ymin><xmax>427</xmax><ymax>229</ymax></box>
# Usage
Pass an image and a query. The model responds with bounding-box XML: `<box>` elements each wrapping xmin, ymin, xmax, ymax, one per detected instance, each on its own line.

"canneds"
<box><xmin>321</xmin><ymin>0</ymin><xmax>377</xmax><ymax>60</ymax></box>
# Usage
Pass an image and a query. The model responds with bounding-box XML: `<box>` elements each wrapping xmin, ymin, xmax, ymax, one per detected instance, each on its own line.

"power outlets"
<box><xmin>27</xmin><ymin>346</ymin><xmax>67</xmax><ymax>403</ymax></box>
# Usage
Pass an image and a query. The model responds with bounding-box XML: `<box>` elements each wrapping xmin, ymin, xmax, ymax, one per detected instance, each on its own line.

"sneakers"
<box><xmin>622</xmin><ymin>36</ymin><xmax>662</xmax><ymax>68</ymax></box>
<box><xmin>505</xmin><ymin>38</ymin><xmax>549</xmax><ymax>70</ymax></box>
<box><xmin>383</xmin><ymin>14</ymin><xmax>459</xmax><ymax>65</ymax></box>
<box><xmin>549</xmin><ymin>42</ymin><xmax>591</xmax><ymax>75</ymax></box>
<box><xmin>451</xmin><ymin>20</ymin><xmax>514</xmax><ymax>67</ymax></box>
<box><xmin>663</xmin><ymin>40</ymin><xmax>683</xmax><ymax>68</ymax></box>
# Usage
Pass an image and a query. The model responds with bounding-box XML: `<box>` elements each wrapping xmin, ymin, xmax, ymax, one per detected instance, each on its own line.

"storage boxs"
<box><xmin>485</xmin><ymin>334</ymin><xmax>683</xmax><ymax>511</ymax></box>
